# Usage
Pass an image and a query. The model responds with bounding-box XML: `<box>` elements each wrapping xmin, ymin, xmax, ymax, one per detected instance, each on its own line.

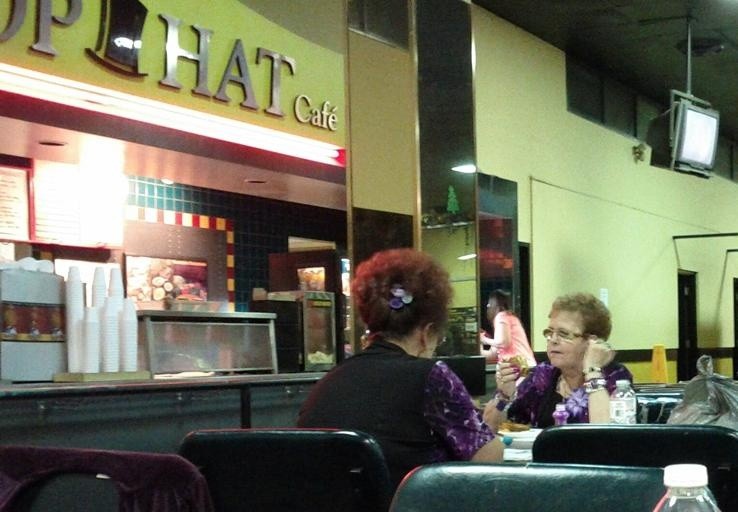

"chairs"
<box><xmin>2</xmin><ymin>452</ymin><xmax>214</xmax><ymax>510</ymax></box>
<box><xmin>390</xmin><ymin>462</ymin><xmax>662</xmax><ymax>512</ymax></box>
<box><xmin>486</xmin><ymin>379</ymin><xmax>735</xmax><ymax>423</ymax></box>
<box><xmin>182</xmin><ymin>429</ymin><xmax>397</xmax><ymax>510</ymax></box>
<box><xmin>532</xmin><ymin>424</ymin><xmax>738</xmax><ymax>508</ymax></box>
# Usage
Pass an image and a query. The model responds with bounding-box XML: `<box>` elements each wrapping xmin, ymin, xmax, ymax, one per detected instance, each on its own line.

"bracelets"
<box><xmin>582</xmin><ymin>367</ymin><xmax>606</xmax><ymax>393</ymax></box>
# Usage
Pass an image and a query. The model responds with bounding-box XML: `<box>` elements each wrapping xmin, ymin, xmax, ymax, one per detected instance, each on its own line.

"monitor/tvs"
<box><xmin>645</xmin><ymin>101</ymin><xmax>721</xmax><ymax>179</ymax></box>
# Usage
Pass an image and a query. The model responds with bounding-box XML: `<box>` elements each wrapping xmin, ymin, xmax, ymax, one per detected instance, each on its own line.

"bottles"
<box><xmin>608</xmin><ymin>380</ymin><xmax>636</xmax><ymax>424</ymax></box>
<box><xmin>551</xmin><ymin>403</ymin><xmax>569</xmax><ymax>425</ymax></box>
<box><xmin>653</xmin><ymin>463</ymin><xmax>721</xmax><ymax>512</ymax></box>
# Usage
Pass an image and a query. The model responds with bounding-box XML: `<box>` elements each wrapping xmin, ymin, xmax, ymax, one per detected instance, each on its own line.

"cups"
<box><xmin>65</xmin><ymin>262</ymin><xmax>139</xmax><ymax>375</ymax></box>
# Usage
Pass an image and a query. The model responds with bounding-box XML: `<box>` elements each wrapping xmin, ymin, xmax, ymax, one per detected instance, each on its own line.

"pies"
<box><xmin>502</xmin><ymin>353</ymin><xmax>527</xmax><ymax>377</ymax></box>
<box><xmin>498</xmin><ymin>423</ymin><xmax>529</xmax><ymax>431</ymax></box>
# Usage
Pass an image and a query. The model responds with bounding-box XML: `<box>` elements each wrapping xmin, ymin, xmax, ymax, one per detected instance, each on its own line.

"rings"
<box><xmin>496</xmin><ymin>371</ymin><xmax>502</xmax><ymax>379</ymax></box>
<box><xmin>501</xmin><ymin>376</ymin><xmax>505</xmax><ymax>383</ymax></box>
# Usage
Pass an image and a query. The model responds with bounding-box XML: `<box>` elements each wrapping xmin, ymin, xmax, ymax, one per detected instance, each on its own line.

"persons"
<box><xmin>480</xmin><ymin>289</ymin><xmax>537</xmax><ymax>397</ymax></box>
<box><xmin>297</xmin><ymin>245</ymin><xmax>506</xmax><ymax>485</ymax></box>
<box><xmin>481</xmin><ymin>292</ymin><xmax>633</xmax><ymax>432</ymax></box>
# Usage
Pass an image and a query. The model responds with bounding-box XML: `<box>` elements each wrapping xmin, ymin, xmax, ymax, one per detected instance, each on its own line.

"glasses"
<box><xmin>543</xmin><ymin>329</ymin><xmax>584</xmax><ymax>340</ymax></box>
